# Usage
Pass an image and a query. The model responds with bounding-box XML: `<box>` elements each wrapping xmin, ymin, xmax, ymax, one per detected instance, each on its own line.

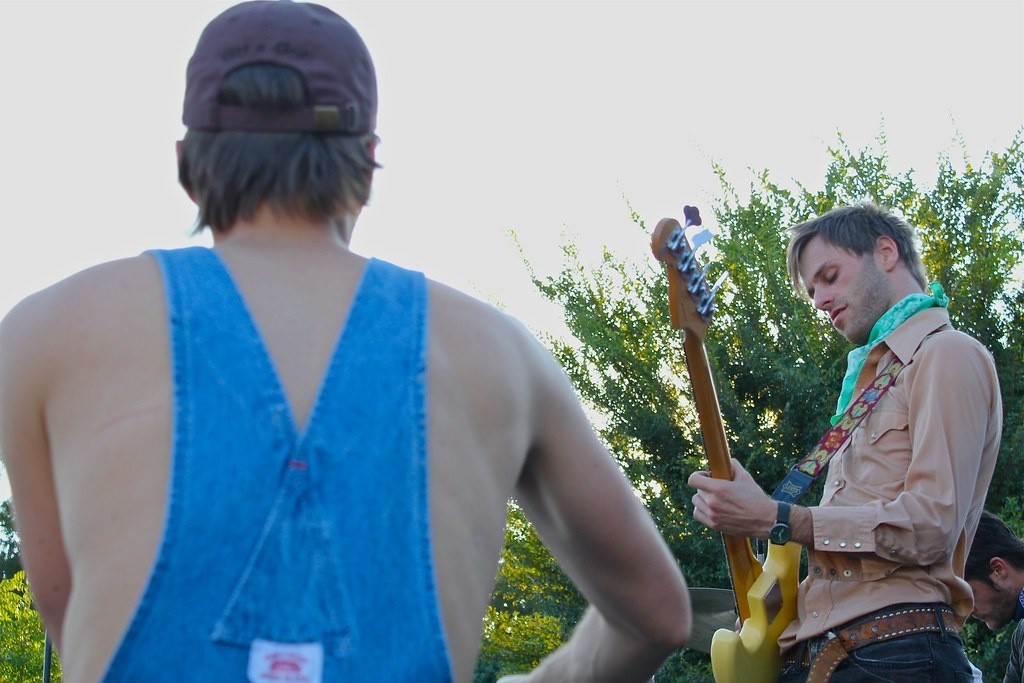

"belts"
<box><xmin>806</xmin><ymin>609</ymin><xmax>959</xmax><ymax>683</ymax></box>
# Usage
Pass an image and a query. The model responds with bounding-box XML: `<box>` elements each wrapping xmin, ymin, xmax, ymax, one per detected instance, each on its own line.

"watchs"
<box><xmin>769</xmin><ymin>501</ymin><xmax>792</xmax><ymax>546</ymax></box>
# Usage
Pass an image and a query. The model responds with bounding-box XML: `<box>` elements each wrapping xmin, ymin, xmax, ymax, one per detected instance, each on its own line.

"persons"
<box><xmin>0</xmin><ymin>0</ymin><xmax>692</xmax><ymax>683</ymax></box>
<box><xmin>688</xmin><ymin>206</ymin><xmax>1002</xmax><ymax>683</ymax></box>
<box><xmin>964</xmin><ymin>509</ymin><xmax>1024</xmax><ymax>683</ymax></box>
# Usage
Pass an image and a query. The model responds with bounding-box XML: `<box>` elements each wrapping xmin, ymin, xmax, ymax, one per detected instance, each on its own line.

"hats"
<box><xmin>181</xmin><ymin>0</ymin><xmax>377</xmax><ymax>135</ymax></box>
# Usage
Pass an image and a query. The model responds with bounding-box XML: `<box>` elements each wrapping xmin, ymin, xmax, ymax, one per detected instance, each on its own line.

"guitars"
<box><xmin>649</xmin><ymin>203</ymin><xmax>804</xmax><ymax>683</ymax></box>
